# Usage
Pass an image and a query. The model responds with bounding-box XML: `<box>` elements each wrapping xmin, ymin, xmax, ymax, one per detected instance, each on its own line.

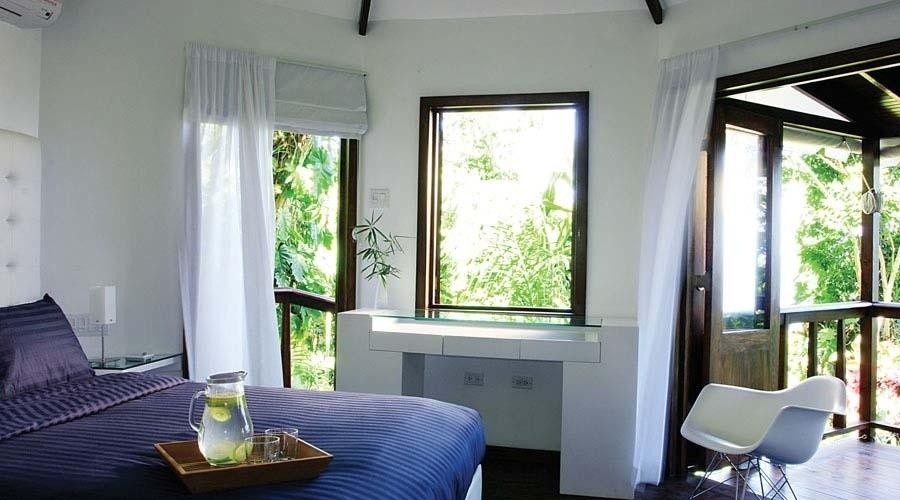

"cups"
<box><xmin>264</xmin><ymin>427</ymin><xmax>299</xmax><ymax>461</ymax></box>
<box><xmin>244</xmin><ymin>435</ymin><xmax>280</xmax><ymax>467</ymax></box>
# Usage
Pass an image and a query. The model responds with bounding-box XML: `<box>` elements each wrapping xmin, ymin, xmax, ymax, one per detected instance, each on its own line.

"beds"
<box><xmin>0</xmin><ymin>380</ymin><xmax>487</xmax><ymax>496</ymax></box>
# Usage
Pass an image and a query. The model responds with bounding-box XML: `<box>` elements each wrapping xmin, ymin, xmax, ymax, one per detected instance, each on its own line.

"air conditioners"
<box><xmin>2</xmin><ymin>2</ymin><xmax>64</xmax><ymax>35</ymax></box>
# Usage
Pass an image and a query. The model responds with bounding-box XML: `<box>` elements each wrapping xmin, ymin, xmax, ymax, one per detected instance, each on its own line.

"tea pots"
<box><xmin>187</xmin><ymin>369</ymin><xmax>253</xmax><ymax>466</ymax></box>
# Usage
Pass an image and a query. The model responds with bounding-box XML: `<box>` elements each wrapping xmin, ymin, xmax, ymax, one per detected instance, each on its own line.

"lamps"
<box><xmin>83</xmin><ymin>282</ymin><xmax>120</xmax><ymax>365</ymax></box>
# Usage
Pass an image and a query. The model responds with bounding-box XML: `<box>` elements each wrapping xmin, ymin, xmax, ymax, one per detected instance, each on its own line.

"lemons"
<box><xmin>207</xmin><ymin>442</ymin><xmax>252</xmax><ymax>466</ymax></box>
<box><xmin>209</xmin><ymin>407</ymin><xmax>232</xmax><ymax>421</ymax></box>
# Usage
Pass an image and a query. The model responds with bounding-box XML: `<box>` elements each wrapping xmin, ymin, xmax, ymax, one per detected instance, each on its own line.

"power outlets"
<box><xmin>461</xmin><ymin>369</ymin><xmax>487</xmax><ymax>392</ymax></box>
<box><xmin>511</xmin><ymin>374</ymin><xmax>532</xmax><ymax>393</ymax></box>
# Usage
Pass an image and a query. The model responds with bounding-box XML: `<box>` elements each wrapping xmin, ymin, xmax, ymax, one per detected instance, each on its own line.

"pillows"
<box><xmin>1</xmin><ymin>293</ymin><xmax>99</xmax><ymax>400</ymax></box>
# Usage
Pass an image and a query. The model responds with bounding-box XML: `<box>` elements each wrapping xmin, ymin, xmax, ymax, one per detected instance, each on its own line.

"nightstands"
<box><xmin>89</xmin><ymin>353</ymin><xmax>181</xmax><ymax>378</ymax></box>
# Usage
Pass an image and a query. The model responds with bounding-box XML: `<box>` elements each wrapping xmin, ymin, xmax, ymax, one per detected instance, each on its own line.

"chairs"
<box><xmin>681</xmin><ymin>373</ymin><xmax>850</xmax><ymax>499</ymax></box>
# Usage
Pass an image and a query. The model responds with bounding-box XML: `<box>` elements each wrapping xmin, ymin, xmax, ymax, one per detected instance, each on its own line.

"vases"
<box><xmin>373</xmin><ymin>271</ymin><xmax>392</xmax><ymax>312</ymax></box>
